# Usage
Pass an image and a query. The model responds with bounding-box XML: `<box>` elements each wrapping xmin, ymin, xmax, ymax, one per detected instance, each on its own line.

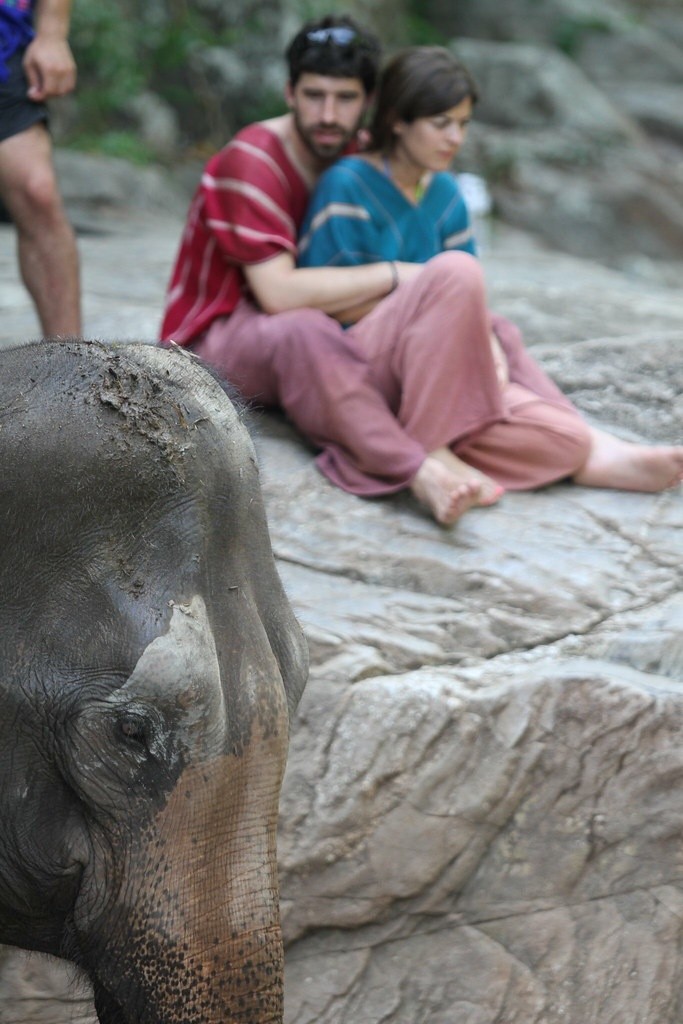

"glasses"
<box><xmin>298</xmin><ymin>28</ymin><xmax>369</xmax><ymax>50</ymax></box>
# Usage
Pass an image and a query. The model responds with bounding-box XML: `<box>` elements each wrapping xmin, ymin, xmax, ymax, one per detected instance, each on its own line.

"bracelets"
<box><xmin>390</xmin><ymin>260</ymin><xmax>399</xmax><ymax>293</ymax></box>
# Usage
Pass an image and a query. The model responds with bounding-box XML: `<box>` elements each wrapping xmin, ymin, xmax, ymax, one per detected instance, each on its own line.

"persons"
<box><xmin>297</xmin><ymin>49</ymin><xmax>593</xmax><ymax>489</ymax></box>
<box><xmin>0</xmin><ymin>0</ymin><xmax>81</xmax><ymax>344</ymax></box>
<box><xmin>160</xmin><ymin>14</ymin><xmax>683</xmax><ymax>527</ymax></box>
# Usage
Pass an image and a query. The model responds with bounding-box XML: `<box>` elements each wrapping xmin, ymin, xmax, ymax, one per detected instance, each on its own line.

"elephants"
<box><xmin>0</xmin><ymin>336</ymin><xmax>309</xmax><ymax>1024</ymax></box>
